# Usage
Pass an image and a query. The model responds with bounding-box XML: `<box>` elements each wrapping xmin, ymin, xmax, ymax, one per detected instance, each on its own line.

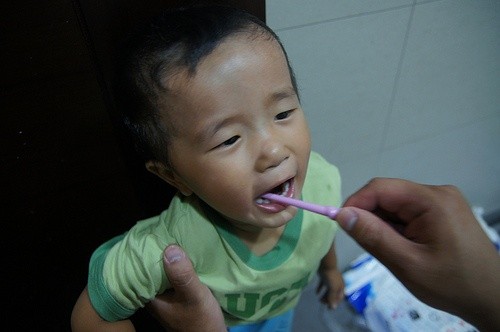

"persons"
<box><xmin>71</xmin><ymin>6</ymin><xmax>346</xmax><ymax>331</ymax></box>
<box><xmin>143</xmin><ymin>177</ymin><xmax>500</xmax><ymax>332</ymax></box>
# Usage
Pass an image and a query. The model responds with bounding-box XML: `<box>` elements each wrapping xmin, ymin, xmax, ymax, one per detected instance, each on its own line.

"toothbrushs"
<box><xmin>261</xmin><ymin>192</ymin><xmax>339</xmax><ymax>220</ymax></box>
